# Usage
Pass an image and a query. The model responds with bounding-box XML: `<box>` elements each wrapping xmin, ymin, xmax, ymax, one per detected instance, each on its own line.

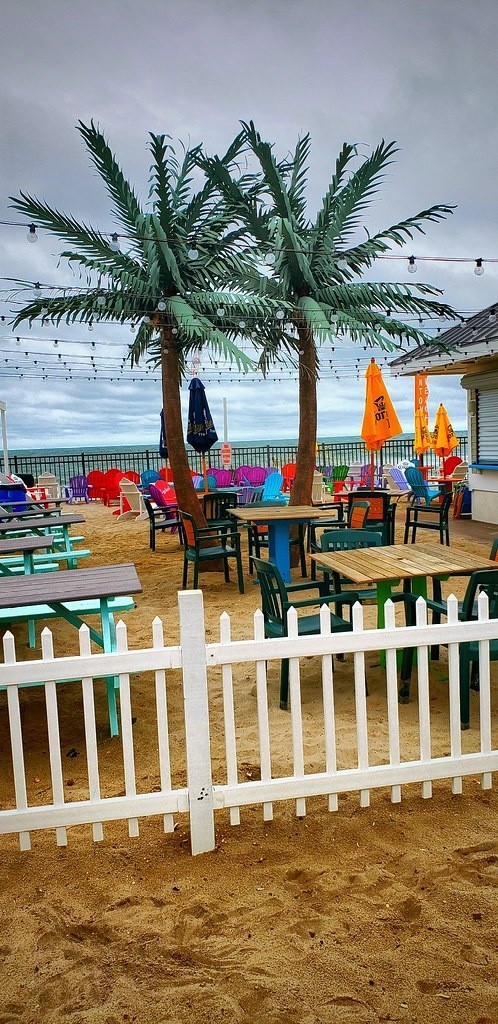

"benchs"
<box><xmin>1</xmin><ymin>597</ymin><xmax>135</xmax><ymax>736</ymax></box>
<box><xmin>0</xmin><ymin>548</ymin><xmax>90</xmax><ymax>570</ymax></box>
<box><xmin>0</xmin><ymin>562</ymin><xmax>60</xmax><ymax>574</ymax></box>
<box><xmin>35</xmin><ymin>535</ymin><xmax>84</xmax><ymax>565</ymax></box>
<box><xmin>35</xmin><ymin>532</ymin><xmax>69</xmax><ymax>538</ymax></box>
<box><xmin>5</xmin><ymin>526</ymin><xmax>69</xmax><ymax>537</ymax></box>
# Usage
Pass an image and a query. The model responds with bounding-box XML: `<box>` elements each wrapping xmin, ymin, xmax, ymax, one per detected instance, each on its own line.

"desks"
<box><xmin>1</xmin><ymin>495</ymin><xmax>71</xmax><ymax>520</ymax></box>
<box><xmin>0</xmin><ymin>506</ymin><xmax>64</xmax><ymax>522</ymax></box>
<box><xmin>307</xmin><ymin>542</ymin><xmax>498</xmax><ymax>665</ymax></box>
<box><xmin>0</xmin><ymin>514</ymin><xmax>85</xmax><ymax>571</ymax></box>
<box><xmin>0</xmin><ymin>562</ymin><xmax>142</xmax><ymax>736</ymax></box>
<box><xmin>331</xmin><ymin>489</ymin><xmax>412</xmax><ymax>538</ymax></box>
<box><xmin>22</xmin><ymin>512</ymin><xmax>74</xmax><ymax>520</ymax></box>
<box><xmin>226</xmin><ymin>505</ymin><xmax>335</xmax><ymax>579</ymax></box>
<box><xmin>426</xmin><ymin>478</ymin><xmax>463</xmax><ymax>505</ymax></box>
<box><xmin>0</xmin><ymin>536</ymin><xmax>55</xmax><ymax>577</ymax></box>
<box><xmin>197</xmin><ymin>492</ymin><xmax>243</xmax><ymax>542</ymax></box>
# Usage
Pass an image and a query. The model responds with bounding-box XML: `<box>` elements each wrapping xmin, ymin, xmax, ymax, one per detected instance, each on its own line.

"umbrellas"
<box><xmin>158</xmin><ymin>408</ymin><xmax>169</xmax><ymax>484</ymax></box>
<box><xmin>413</xmin><ymin>403</ymin><xmax>460</xmax><ymax>479</ymax></box>
<box><xmin>361</xmin><ymin>357</ymin><xmax>403</xmax><ymax>491</ymax></box>
<box><xmin>186</xmin><ymin>378</ymin><xmax>219</xmax><ymax>492</ymax></box>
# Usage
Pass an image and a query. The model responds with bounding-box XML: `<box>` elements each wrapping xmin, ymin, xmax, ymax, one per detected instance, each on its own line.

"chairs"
<box><xmin>0</xmin><ymin>455</ymin><xmax>498</xmax><ymax>737</ymax></box>
